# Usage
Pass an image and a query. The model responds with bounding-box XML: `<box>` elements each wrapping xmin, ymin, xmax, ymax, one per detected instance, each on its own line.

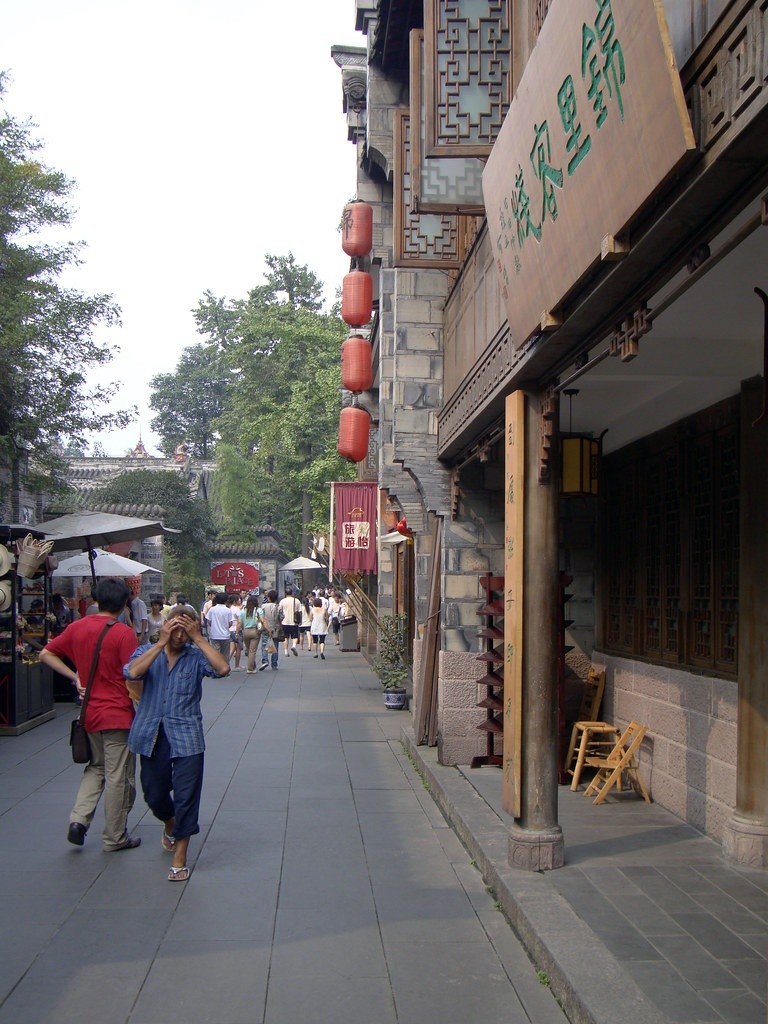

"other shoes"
<box><xmin>68</xmin><ymin>821</ymin><xmax>85</xmax><ymax>847</ymax></box>
<box><xmin>247</xmin><ymin>670</ymin><xmax>258</xmax><ymax>674</ymax></box>
<box><xmin>320</xmin><ymin>653</ymin><xmax>325</xmax><ymax>660</ymax></box>
<box><xmin>291</xmin><ymin>646</ymin><xmax>300</xmax><ymax>656</ymax></box>
<box><xmin>259</xmin><ymin>662</ymin><xmax>269</xmax><ymax>671</ymax></box>
<box><xmin>234</xmin><ymin>666</ymin><xmax>245</xmax><ymax>671</ymax></box>
<box><xmin>121</xmin><ymin>836</ymin><xmax>141</xmax><ymax>848</ymax></box>
<box><xmin>313</xmin><ymin>654</ymin><xmax>318</xmax><ymax>658</ymax></box>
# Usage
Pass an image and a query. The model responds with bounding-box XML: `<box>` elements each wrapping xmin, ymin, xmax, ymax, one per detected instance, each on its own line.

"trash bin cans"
<box><xmin>338</xmin><ymin>614</ymin><xmax>360</xmax><ymax>652</ymax></box>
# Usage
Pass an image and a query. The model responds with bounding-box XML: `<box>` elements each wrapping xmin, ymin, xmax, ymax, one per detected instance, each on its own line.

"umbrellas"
<box><xmin>32</xmin><ymin>509</ymin><xmax>183</xmax><ymax>592</ymax></box>
<box><xmin>277</xmin><ymin>556</ymin><xmax>327</xmax><ymax>590</ymax></box>
<box><xmin>50</xmin><ymin>547</ymin><xmax>166</xmax><ymax>579</ymax></box>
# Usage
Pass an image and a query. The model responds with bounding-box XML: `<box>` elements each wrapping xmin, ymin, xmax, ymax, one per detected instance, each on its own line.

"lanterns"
<box><xmin>340</xmin><ymin>197</ymin><xmax>372</xmax><ymax>463</ymax></box>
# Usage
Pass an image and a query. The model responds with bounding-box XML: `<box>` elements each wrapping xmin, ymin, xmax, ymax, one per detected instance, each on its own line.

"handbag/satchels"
<box><xmin>67</xmin><ymin>714</ymin><xmax>88</xmax><ymax>763</ymax></box>
<box><xmin>256</xmin><ymin>606</ymin><xmax>265</xmax><ymax>633</ymax></box>
<box><xmin>265</xmin><ymin>636</ymin><xmax>277</xmax><ymax>654</ymax></box>
<box><xmin>294</xmin><ymin>612</ymin><xmax>301</xmax><ymax>624</ymax></box>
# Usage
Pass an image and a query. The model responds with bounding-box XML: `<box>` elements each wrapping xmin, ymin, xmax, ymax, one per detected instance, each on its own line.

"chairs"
<box><xmin>583</xmin><ymin>721</ymin><xmax>651</xmax><ymax>805</ymax></box>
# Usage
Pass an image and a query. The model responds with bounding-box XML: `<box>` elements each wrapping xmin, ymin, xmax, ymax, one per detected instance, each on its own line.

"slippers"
<box><xmin>168</xmin><ymin>863</ymin><xmax>190</xmax><ymax>881</ymax></box>
<box><xmin>161</xmin><ymin>820</ymin><xmax>177</xmax><ymax>851</ymax></box>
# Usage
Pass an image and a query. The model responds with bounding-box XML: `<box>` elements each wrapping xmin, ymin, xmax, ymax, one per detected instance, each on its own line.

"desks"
<box><xmin>564</xmin><ymin>720</ymin><xmax>624</xmax><ymax>791</ymax></box>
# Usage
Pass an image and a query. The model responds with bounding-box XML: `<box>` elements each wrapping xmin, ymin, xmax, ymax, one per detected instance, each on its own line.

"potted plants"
<box><xmin>377</xmin><ymin>612</ymin><xmax>411</xmax><ymax>709</ymax></box>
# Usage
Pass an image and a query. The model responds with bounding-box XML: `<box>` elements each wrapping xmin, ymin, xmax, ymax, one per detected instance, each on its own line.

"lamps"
<box><xmin>557</xmin><ymin>389</ymin><xmax>609</xmax><ymax>500</ymax></box>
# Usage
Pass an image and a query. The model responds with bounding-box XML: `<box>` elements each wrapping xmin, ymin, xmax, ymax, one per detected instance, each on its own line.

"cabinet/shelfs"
<box><xmin>467</xmin><ymin>568</ymin><xmax>580</xmax><ymax>769</ymax></box>
<box><xmin>0</xmin><ymin>524</ymin><xmax>58</xmax><ymax>727</ymax></box>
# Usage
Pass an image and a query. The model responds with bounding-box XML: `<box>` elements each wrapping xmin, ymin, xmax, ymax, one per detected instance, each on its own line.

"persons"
<box><xmin>122</xmin><ymin>605</ymin><xmax>231</xmax><ymax>881</ymax></box>
<box><xmin>37</xmin><ymin>585</ymin><xmax>355</xmax><ymax>705</ymax></box>
<box><xmin>39</xmin><ymin>577</ymin><xmax>142</xmax><ymax>853</ymax></box>
